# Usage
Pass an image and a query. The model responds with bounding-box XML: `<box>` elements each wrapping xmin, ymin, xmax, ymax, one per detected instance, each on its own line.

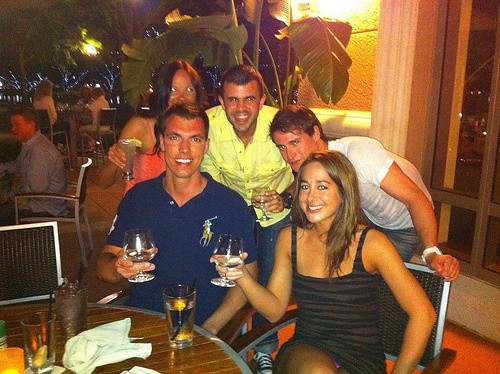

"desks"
<box><xmin>56</xmin><ymin>107</ymin><xmax>92</xmax><ymax>168</ymax></box>
<box><xmin>0</xmin><ymin>303</ymin><xmax>251</xmax><ymax>374</ymax></box>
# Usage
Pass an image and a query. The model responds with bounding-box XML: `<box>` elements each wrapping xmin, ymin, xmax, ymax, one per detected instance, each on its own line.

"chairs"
<box><xmin>14</xmin><ymin>156</ymin><xmax>95</xmax><ymax>269</ymax></box>
<box><xmin>100</xmin><ymin>212</ymin><xmax>257</xmax><ymax>364</ymax></box>
<box><xmin>231</xmin><ymin>259</ymin><xmax>458</xmax><ymax>374</ymax></box>
<box><xmin>0</xmin><ymin>220</ymin><xmax>62</xmax><ymax>304</ymax></box>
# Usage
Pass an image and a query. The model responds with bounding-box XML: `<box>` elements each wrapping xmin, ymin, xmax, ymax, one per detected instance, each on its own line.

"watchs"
<box><xmin>421</xmin><ymin>246</ymin><xmax>442</xmax><ymax>263</ymax></box>
<box><xmin>280</xmin><ymin>192</ymin><xmax>293</xmax><ymax>208</ymax></box>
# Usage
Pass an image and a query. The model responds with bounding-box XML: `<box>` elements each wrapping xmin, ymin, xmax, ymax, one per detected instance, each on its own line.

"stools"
<box><xmin>33</xmin><ymin>108</ymin><xmax>70</xmax><ymax>169</ymax></box>
<box><xmin>80</xmin><ymin>107</ymin><xmax>117</xmax><ymax>168</ymax></box>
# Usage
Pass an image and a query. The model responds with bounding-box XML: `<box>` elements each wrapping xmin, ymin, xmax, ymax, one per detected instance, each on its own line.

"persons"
<box><xmin>268</xmin><ymin>105</ymin><xmax>461</xmax><ymax>282</ymax></box>
<box><xmin>77</xmin><ymin>85</ymin><xmax>110</xmax><ymax>152</ymax></box>
<box><xmin>98</xmin><ymin>61</ymin><xmax>207</xmax><ymax>201</ymax></box>
<box><xmin>94</xmin><ymin>101</ymin><xmax>259</xmax><ymax>336</ymax></box>
<box><xmin>202</xmin><ymin>65</ymin><xmax>298</xmax><ymax>374</ymax></box>
<box><xmin>209</xmin><ymin>149</ymin><xmax>437</xmax><ymax>374</ymax></box>
<box><xmin>33</xmin><ymin>80</ymin><xmax>69</xmax><ymax>143</ymax></box>
<box><xmin>0</xmin><ymin>106</ymin><xmax>66</xmax><ymax>226</ymax></box>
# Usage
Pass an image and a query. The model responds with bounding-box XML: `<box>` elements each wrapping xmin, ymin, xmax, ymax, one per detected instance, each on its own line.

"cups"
<box><xmin>162</xmin><ymin>284</ymin><xmax>197</xmax><ymax>349</ymax></box>
<box><xmin>0</xmin><ymin>347</ymin><xmax>25</xmax><ymax>374</ymax></box>
<box><xmin>117</xmin><ymin>139</ymin><xmax>138</xmax><ymax>181</ymax></box>
<box><xmin>55</xmin><ymin>282</ymin><xmax>88</xmax><ymax>339</ymax></box>
<box><xmin>20</xmin><ymin>312</ymin><xmax>56</xmax><ymax>374</ymax></box>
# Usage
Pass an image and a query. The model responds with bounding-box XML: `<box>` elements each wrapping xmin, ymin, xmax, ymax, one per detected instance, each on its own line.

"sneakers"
<box><xmin>253</xmin><ymin>351</ymin><xmax>274</xmax><ymax>374</ymax></box>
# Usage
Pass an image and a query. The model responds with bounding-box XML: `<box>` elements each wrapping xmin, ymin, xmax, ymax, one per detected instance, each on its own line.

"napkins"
<box><xmin>62</xmin><ymin>317</ymin><xmax>152</xmax><ymax>374</ymax></box>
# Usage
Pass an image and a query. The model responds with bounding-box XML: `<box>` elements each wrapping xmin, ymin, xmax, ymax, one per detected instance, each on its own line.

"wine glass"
<box><xmin>251</xmin><ymin>186</ymin><xmax>274</xmax><ymax>222</ymax></box>
<box><xmin>210</xmin><ymin>234</ymin><xmax>243</xmax><ymax>287</ymax></box>
<box><xmin>123</xmin><ymin>228</ymin><xmax>156</xmax><ymax>283</ymax></box>
